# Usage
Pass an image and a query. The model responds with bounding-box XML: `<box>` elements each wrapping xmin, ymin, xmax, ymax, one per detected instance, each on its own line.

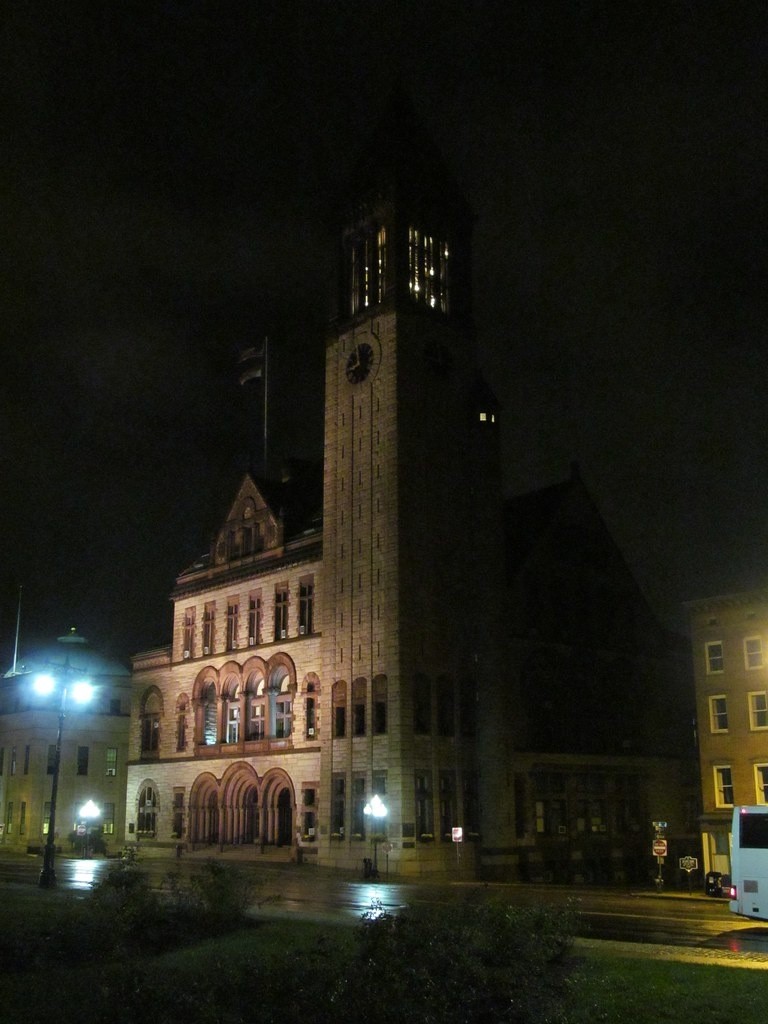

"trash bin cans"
<box><xmin>363</xmin><ymin>858</ymin><xmax>372</xmax><ymax>877</ymax></box>
<box><xmin>705</xmin><ymin>871</ymin><xmax>721</xmax><ymax>898</ymax></box>
<box><xmin>83</xmin><ymin>847</ymin><xmax>94</xmax><ymax>859</ymax></box>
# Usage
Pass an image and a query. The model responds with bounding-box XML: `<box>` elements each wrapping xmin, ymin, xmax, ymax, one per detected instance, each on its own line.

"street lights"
<box><xmin>26</xmin><ymin>646</ymin><xmax>96</xmax><ymax>887</ymax></box>
<box><xmin>363</xmin><ymin>794</ymin><xmax>388</xmax><ymax>878</ymax></box>
<box><xmin>79</xmin><ymin>799</ymin><xmax>100</xmax><ymax>859</ymax></box>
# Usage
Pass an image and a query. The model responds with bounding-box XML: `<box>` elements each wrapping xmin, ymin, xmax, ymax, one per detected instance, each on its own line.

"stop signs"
<box><xmin>451</xmin><ymin>827</ymin><xmax>463</xmax><ymax>843</ymax></box>
<box><xmin>652</xmin><ymin>839</ymin><xmax>668</xmax><ymax>857</ymax></box>
<box><xmin>76</xmin><ymin>825</ymin><xmax>86</xmax><ymax>836</ymax></box>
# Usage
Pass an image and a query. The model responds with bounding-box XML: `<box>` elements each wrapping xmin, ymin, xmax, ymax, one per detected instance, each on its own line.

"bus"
<box><xmin>727</xmin><ymin>804</ymin><xmax>768</xmax><ymax>923</ymax></box>
